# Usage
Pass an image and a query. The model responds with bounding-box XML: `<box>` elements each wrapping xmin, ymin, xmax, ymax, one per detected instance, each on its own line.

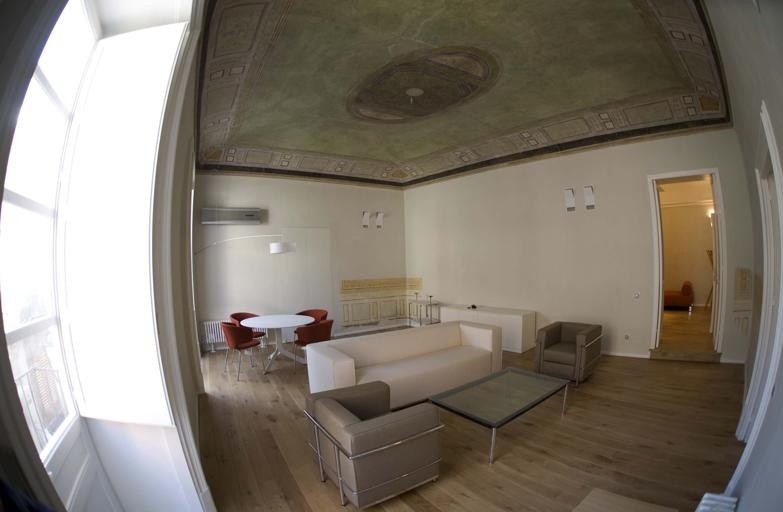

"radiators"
<box><xmin>202</xmin><ymin>318</ymin><xmax>269</xmax><ymax>354</ymax></box>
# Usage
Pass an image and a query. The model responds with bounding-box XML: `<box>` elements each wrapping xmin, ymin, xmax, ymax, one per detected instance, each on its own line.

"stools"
<box><xmin>663</xmin><ymin>279</ymin><xmax>695</xmax><ymax>313</ymax></box>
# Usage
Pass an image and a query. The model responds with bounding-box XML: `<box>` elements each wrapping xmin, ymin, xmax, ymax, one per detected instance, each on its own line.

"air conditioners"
<box><xmin>199</xmin><ymin>207</ymin><xmax>263</xmax><ymax>228</ymax></box>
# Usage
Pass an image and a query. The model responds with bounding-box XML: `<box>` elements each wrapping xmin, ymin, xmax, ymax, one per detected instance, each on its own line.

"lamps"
<box><xmin>193</xmin><ymin>233</ymin><xmax>292</xmax><ymax>256</ymax></box>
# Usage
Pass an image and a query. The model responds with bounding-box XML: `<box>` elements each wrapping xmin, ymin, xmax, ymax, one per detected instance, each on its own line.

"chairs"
<box><xmin>304</xmin><ymin>381</ymin><xmax>449</xmax><ymax>512</ymax></box>
<box><xmin>536</xmin><ymin>320</ymin><xmax>603</xmax><ymax>390</ymax></box>
<box><xmin>219</xmin><ymin>309</ymin><xmax>334</xmax><ymax>383</ymax></box>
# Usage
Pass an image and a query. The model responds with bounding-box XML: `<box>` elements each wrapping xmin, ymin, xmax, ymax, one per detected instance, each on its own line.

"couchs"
<box><xmin>304</xmin><ymin>319</ymin><xmax>503</xmax><ymax>412</ymax></box>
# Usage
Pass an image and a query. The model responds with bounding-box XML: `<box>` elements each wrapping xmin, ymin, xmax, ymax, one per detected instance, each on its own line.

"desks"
<box><xmin>439</xmin><ymin>302</ymin><xmax>538</xmax><ymax>355</ymax></box>
